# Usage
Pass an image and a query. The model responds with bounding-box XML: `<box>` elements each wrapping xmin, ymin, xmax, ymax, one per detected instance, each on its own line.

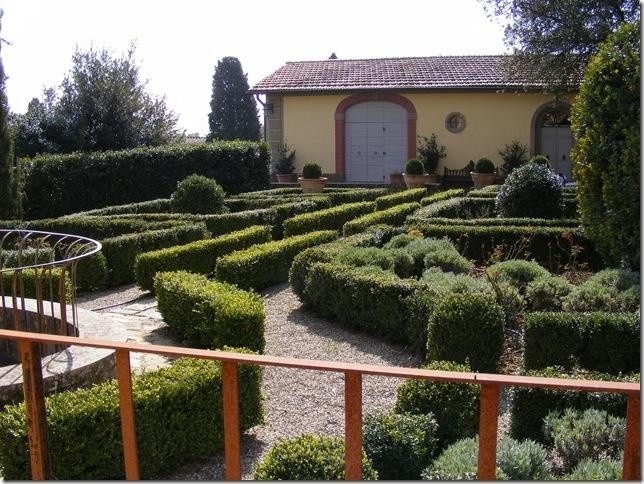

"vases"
<box><xmin>298</xmin><ymin>160</ymin><xmax>328</xmax><ymax>193</ymax></box>
<box><xmin>274</xmin><ymin>140</ymin><xmax>298</xmax><ymax>183</ymax></box>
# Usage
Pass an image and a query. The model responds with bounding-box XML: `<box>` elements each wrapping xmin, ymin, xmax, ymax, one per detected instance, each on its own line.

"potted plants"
<box><xmin>401</xmin><ymin>159</ymin><xmax>429</xmax><ymax>188</ymax></box>
<box><xmin>416</xmin><ymin>132</ymin><xmax>447</xmax><ymax>182</ymax></box>
<box><xmin>470</xmin><ymin>157</ymin><xmax>497</xmax><ymax>190</ymax></box>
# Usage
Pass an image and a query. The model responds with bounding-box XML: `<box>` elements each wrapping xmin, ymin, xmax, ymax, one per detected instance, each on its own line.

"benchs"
<box><xmin>443</xmin><ymin>166</ymin><xmax>499</xmax><ymax>191</ymax></box>
